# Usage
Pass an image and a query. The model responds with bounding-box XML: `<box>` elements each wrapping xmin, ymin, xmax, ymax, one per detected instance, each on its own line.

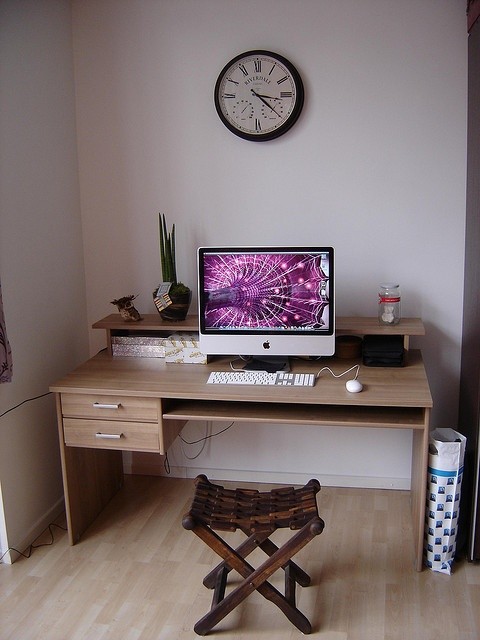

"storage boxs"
<box><xmin>111</xmin><ymin>336</ymin><xmax>166</xmax><ymax>358</ymax></box>
<box><xmin>164</xmin><ymin>333</ymin><xmax>207</xmax><ymax>365</ymax></box>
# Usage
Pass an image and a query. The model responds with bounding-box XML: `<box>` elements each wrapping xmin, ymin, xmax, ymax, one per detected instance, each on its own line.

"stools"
<box><xmin>182</xmin><ymin>473</ymin><xmax>325</xmax><ymax>634</ymax></box>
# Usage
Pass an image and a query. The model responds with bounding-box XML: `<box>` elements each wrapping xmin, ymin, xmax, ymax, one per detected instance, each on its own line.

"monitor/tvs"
<box><xmin>195</xmin><ymin>246</ymin><xmax>337</xmax><ymax>373</ymax></box>
<box><xmin>206</xmin><ymin>370</ymin><xmax>317</xmax><ymax>387</ymax></box>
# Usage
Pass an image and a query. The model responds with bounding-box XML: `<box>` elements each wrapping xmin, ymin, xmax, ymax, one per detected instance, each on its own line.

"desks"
<box><xmin>49</xmin><ymin>313</ymin><xmax>435</xmax><ymax>573</ymax></box>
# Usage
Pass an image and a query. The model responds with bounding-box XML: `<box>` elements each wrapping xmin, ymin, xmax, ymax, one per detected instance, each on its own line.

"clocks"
<box><xmin>214</xmin><ymin>51</ymin><xmax>304</xmax><ymax>143</ymax></box>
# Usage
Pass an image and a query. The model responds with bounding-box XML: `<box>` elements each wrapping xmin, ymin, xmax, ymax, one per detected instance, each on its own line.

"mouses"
<box><xmin>344</xmin><ymin>380</ymin><xmax>363</xmax><ymax>392</ymax></box>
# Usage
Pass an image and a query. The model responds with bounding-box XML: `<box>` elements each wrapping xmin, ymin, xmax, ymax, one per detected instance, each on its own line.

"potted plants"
<box><xmin>153</xmin><ymin>212</ymin><xmax>190</xmax><ymax>323</ymax></box>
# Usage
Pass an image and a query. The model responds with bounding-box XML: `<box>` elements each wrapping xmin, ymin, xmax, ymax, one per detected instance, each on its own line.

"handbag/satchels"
<box><xmin>425</xmin><ymin>428</ymin><xmax>467</xmax><ymax>576</ymax></box>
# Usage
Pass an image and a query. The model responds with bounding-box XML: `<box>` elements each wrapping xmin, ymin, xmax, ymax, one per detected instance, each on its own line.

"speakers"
<box><xmin>365</xmin><ymin>335</ymin><xmax>405</xmax><ymax>367</ymax></box>
